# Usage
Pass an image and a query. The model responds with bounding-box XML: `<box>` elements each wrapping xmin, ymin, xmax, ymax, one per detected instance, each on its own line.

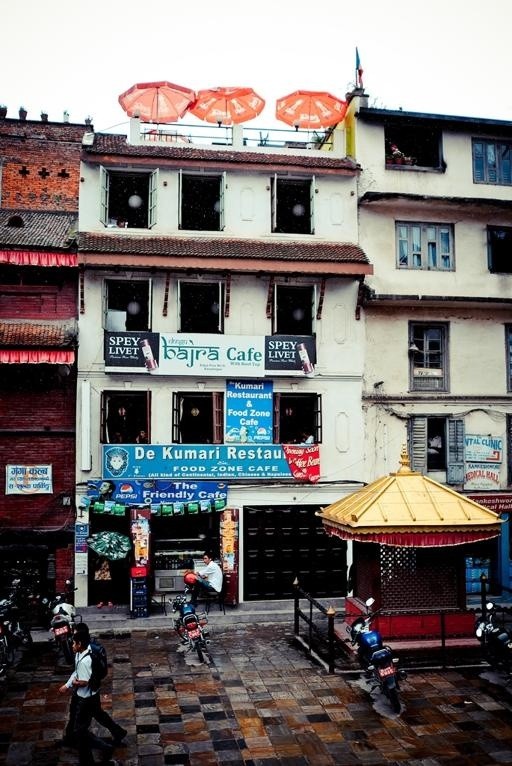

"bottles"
<box><xmin>154</xmin><ymin>556</ymin><xmax>193</xmax><ymax>570</ymax></box>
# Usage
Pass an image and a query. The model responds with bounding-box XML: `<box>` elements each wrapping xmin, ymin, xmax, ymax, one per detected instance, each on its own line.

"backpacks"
<box><xmin>87</xmin><ymin>635</ymin><xmax>108</xmax><ymax>678</ymax></box>
<box><xmin>74</xmin><ymin>651</ymin><xmax>104</xmax><ymax>691</ymax></box>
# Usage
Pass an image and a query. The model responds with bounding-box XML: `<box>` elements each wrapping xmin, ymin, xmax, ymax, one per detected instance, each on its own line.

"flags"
<box><xmin>356</xmin><ymin>49</ymin><xmax>364</xmax><ymax>91</ymax></box>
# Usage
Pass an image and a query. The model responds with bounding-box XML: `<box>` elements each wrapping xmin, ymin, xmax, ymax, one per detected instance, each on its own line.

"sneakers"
<box><xmin>111</xmin><ymin>729</ymin><xmax>127</xmax><ymax>745</ymax></box>
<box><xmin>96</xmin><ymin>601</ymin><xmax>114</xmax><ymax>608</ymax></box>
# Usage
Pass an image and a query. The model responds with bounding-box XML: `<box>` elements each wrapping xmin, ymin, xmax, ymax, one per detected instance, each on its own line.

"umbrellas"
<box><xmin>187</xmin><ymin>85</ymin><xmax>266</xmax><ymax>146</ymax></box>
<box><xmin>276</xmin><ymin>89</ymin><xmax>348</xmax><ymax>148</ymax></box>
<box><xmin>85</xmin><ymin>530</ymin><xmax>132</xmax><ymax>562</ymax></box>
<box><xmin>117</xmin><ymin>80</ymin><xmax>196</xmax><ymax>131</ymax></box>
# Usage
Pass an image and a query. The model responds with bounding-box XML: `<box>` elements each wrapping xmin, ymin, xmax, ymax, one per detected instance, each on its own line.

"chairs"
<box><xmin>199</xmin><ymin>573</ymin><xmax>229</xmax><ymax>615</ymax></box>
<box><xmin>148</xmin><ymin>579</ymin><xmax>167</xmax><ymax>614</ymax></box>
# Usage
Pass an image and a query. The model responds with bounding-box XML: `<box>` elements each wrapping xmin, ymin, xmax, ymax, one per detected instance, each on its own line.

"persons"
<box><xmin>183</xmin><ymin>551</ymin><xmax>224</xmax><ymax>608</ymax></box>
<box><xmin>346</xmin><ymin>550</ymin><xmax>376</xmax><ymax>603</ymax></box>
<box><xmin>92</xmin><ymin>553</ymin><xmax>115</xmax><ymax>609</ymax></box>
<box><xmin>89</xmin><ymin>481</ymin><xmax>120</xmax><ymax>505</ymax></box>
<box><xmin>52</xmin><ymin>622</ymin><xmax>128</xmax><ymax>765</ymax></box>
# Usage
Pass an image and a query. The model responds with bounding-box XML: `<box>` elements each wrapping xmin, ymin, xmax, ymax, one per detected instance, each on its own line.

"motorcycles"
<box><xmin>168</xmin><ymin>586</ymin><xmax>211</xmax><ymax>662</ymax></box>
<box><xmin>0</xmin><ymin>568</ymin><xmax>41</xmax><ymax>682</ymax></box>
<box><xmin>473</xmin><ymin>600</ymin><xmax>512</xmax><ymax>672</ymax></box>
<box><xmin>345</xmin><ymin>597</ymin><xmax>407</xmax><ymax>712</ymax></box>
<box><xmin>38</xmin><ymin>580</ymin><xmax>83</xmax><ymax>672</ymax></box>
